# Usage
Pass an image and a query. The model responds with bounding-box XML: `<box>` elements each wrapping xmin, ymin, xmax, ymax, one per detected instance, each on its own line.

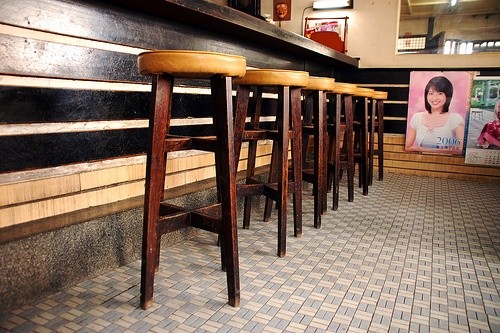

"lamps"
<box><xmin>301</xmin><ymin>0</ymin><xmax>354</xmax><ymax>36</ymax></box>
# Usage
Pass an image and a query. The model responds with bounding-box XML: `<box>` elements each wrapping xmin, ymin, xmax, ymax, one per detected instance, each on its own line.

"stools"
<box><xmin>137</xmin><ymin>50</ymin><xmax>247</xmax><ymax>310</ymax></box>
<box><xmin>263</xmin><ymin>75</ymin><xmax>335</xmax><ymax>237</ymax></box>
<box><xmin>216</xmin><ymin>68</ymin><xmax>311</xmax><ymax>259</ymax></box>
<box><xmin>337</xmin><ymin>86</ymin><xmax>375</xmax><ymax>198</ymax></box>
<box><xmin>338</xmin><ymin>90</ymin><xmax>388</xmax><ymax>188</ymax></box>
<box><xmin>289</xmin><ymin>81</ymin><xmax>357</xmax><ymax>211</ymax></box>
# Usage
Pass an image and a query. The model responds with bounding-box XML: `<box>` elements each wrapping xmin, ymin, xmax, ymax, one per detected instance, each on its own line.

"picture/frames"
<box><xmin>272</xmin><ymin>0</ymin><xmax>291</xmax><ymax>21</ymax></box>
<box><xmin>227</xmin><ymin>0</ymin><xmax>261</xmax><ymax>15</ymax></box>
<box><xmin>303</xmin><ymin>16</ymin><xmax>349</xmax><ymax>55</ymax></box>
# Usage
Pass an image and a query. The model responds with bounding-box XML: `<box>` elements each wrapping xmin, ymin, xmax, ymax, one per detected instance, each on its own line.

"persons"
<box><xmin>406</xmin><ymin>75</ymin><xmax>465</xmax><ymax>153</ymax></box>
<box><xmin>476</xmin><ymin>100</ymin><xmax>500</xmax><ymax>149</ymax></box>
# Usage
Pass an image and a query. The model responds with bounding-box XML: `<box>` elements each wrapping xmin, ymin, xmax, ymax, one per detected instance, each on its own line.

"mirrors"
<box><xmin>394</xmin><ymin>0</ymin><xmax>498</xmax><ymax>55</ymax></box>
